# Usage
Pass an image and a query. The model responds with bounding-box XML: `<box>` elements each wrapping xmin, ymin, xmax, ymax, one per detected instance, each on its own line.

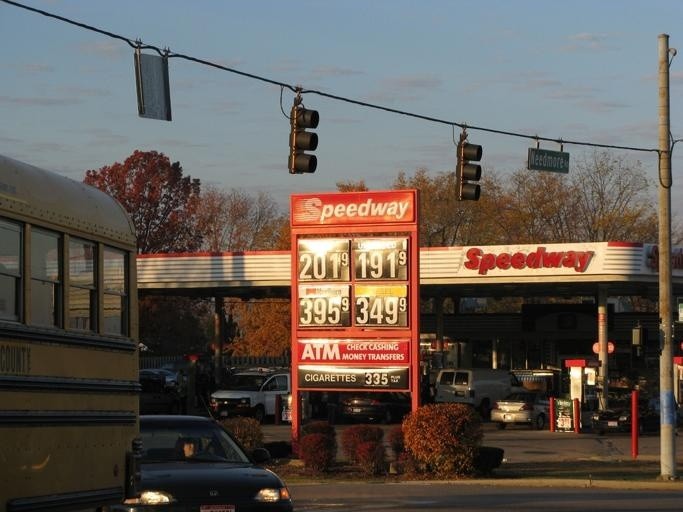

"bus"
<box><xmin>0</xmin><ymin>151</ymin><xmax>140</xmax><ymax>510</ymax></box>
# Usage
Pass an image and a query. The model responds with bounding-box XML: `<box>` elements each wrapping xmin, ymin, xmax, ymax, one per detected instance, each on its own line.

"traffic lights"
<box><xmin>456</xmin><ymin>140</ymin><xmax>483</xmax><ymax>201</ymax></box>
<box><xmin>290</xmin><ymin>107</ymin><xmax>319</xmax><ymax>174</ymax></box>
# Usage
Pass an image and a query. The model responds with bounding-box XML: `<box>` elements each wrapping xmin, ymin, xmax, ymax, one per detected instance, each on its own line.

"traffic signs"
<box><xmin>527</xmin><ymin>148</ymin><xmax>569</xmax><ymax>174</ymax></box>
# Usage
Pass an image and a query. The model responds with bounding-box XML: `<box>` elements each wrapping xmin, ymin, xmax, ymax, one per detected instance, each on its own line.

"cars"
<box><xmin>589</xmin><ymin>398</ymin><xmax>660</xmax><ymax>435</ymax></box>
<box><xmin>139</xmin><ymin>413</ymin><xmax>294</xmax><ymax>510</ymax></box>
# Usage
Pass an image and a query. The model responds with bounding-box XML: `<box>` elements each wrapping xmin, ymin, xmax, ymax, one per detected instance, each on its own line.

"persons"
<box><xmin>181</xmin><ymin>436</ymin><xmax>201</xmax><ymax>457</ymax></box>
